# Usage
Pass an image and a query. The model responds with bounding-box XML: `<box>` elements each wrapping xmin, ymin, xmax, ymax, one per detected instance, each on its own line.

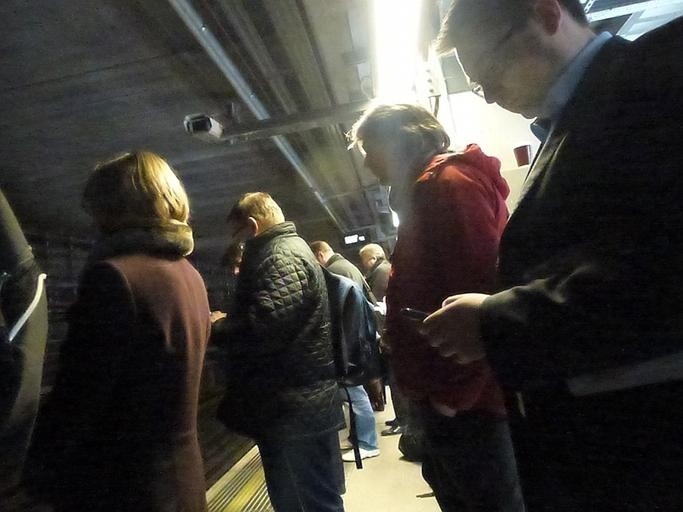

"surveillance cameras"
<box><xmin>183</xmin><ymin>114</ymin><xmax>224</xmax><ymax>143</ymax></box>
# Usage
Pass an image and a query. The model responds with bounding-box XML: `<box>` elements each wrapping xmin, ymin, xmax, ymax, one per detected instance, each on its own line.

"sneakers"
<box><xmin>339</xmin><ymin>438</ymin><xmax>353</xmax><ymax>449</ymax></box>
<box><xmin>342</xmin><ymin>447</ymin><xmax>380</xmax><ymax>462</ymax></box>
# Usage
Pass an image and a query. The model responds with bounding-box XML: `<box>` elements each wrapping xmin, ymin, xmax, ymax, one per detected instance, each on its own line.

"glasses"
<box><xmin>471</xmin><ymin>25</ymin><xmax>518</xmax><ymax>97</ymax></box>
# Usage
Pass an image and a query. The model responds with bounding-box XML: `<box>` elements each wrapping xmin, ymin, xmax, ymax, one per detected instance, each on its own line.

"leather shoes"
<box><xmin>381</xmin><ymin>418</ymin><xmax>402</xmax><ymax>436</ymax></box>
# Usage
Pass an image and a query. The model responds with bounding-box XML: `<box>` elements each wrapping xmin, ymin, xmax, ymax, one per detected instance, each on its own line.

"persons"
<box><xmin>344</xmin><ymin>103</ymin><xmax>528</xmax><ymax>510</ymax></box>
<box><xmin>417</xmin><ymin>0</ymin><xmax>681</xmax><ymax>511</ymax></box>
<box><xmin>30</xmin><ymin>150</ymin><xmax>214</xmax><ymax>509</ymax></box>
<box><xmin>359</xmin><ymin>244</ymin><xmax>408</xmax><ymax>436</ymax></box>
<box><xmin>209</xmin><ymin>193</ymin><xmax>346</xmax><ymax>510</ymax></box>
<box><xmin>308</xmin><ymin>241</ymin><xmax>379</xmax><ymax>461</ymax></box>
<box><xmin>222</xmin><ymin>241</ymin><xmax>344</xmax><ymax>495</ymax></box>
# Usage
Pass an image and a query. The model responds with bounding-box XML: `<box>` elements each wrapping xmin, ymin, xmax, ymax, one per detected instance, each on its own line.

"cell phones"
<box><xmin>396</xmin><ymin>307</ymin><xmax>429</xmax><ymax>322</ymax></box>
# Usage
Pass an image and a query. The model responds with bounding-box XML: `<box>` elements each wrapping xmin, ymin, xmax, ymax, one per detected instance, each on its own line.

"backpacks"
<box><xmin>319</xmin><ymin>263</ymin><xmax>383</xmax><ymax>387</ymax></box>
